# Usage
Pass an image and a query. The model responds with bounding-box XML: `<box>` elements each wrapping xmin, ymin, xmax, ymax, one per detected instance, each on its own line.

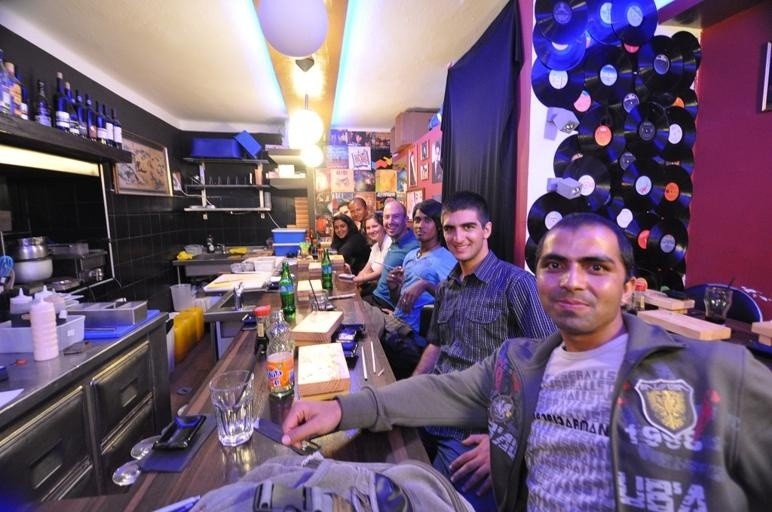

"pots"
<box><xmin>8</xmin><ymin>235</ymin><xmax>49</xmax><ymax>261</ymax></box>
<box><xmin>13</xmin><ymin>254</ymin><xmax>54</xmax><ymax>283</ymax></box>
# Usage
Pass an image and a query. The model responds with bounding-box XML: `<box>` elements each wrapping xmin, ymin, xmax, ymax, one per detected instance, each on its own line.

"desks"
<box><xmin>628</xmin><ymin>300</ymin><xmax>771</xmax><ymax>372</ymax></box>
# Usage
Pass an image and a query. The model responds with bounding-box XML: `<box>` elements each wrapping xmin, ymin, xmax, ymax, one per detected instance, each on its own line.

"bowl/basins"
<box><xmin>183</xmin><ymin>243</ymin><xmax>205</xmax><ymax>256</ymax></box>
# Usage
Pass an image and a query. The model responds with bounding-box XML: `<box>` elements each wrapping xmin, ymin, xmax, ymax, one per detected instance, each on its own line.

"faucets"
<box><xmin>216</xmin><ymin>242</ymin><xmax>227</xmax><ymax>254</ymax></box>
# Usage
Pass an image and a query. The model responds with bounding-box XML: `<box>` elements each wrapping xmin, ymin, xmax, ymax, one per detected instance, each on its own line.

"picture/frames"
<box><xmin>419</xmin><ymin>162</ymin><xmax>430</xmax><ymax>181</ymax></box>
<box><xmin>403</xmin><ymin>144</ymin><xmax>419</xmax><ymax>189</ymax></box>
<box><xmin>405</xmin><ymin>187</ymin><xmax>425</xmax><ymax>224</ymax></box>
<box><xmin>420</xmin><ymin>139</ymin><xmax>429</xmax><ymax>161</ymax></box>
<box><xmin>109</xmin><ymin>124</ymin><xmax>174</xmax><ymax>198</ymax></box>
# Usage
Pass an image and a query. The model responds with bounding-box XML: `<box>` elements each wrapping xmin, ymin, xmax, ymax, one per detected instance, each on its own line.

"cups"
<box><xmin>703</xmin><ymin>286</ymin><xmax>734</xmax><ymax>327</ymax></box>
<box><xmin>208</xmin><ymin>369</ymin><xmax>256</xmax><ymax>447</ymax></box>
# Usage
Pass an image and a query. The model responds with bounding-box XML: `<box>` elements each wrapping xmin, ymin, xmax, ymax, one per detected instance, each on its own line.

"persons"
<box><xmin>431</xmin><ymin>141</ymin><xmax>443</xmax><ymax>181</ymax></box>
<box><xmin>409</xmin><ymin>152</ymin><xmax>417</xmax><ymax>187</ymax></box>
<box><xmin>329</xmin><ymin>191</ymin><xmax>558</xmax><ymax>512</ymax></box>
<box><xmin>373</xmin><ymin>134</ymin><xmax>389</xmax><ymax>146</ymax></box>
<box><xmin>117</xmin><ymin>140</ymin><xmax>168</xmax><ymax>192</ymax></box>
<box><xmin>354</xmin><ymin>132</ymin><xmax>364</xmax><ymax>145</ymax></box>
<box><xmin>279</xmin><ymin>214</ymin><xmax>771</xmax><ymax>512</ymax></box>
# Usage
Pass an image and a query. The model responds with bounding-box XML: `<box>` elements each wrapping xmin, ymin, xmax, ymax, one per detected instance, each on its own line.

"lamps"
<box><xmin>284</xmin><ymin>56</ymin><xmax>330</xmax><ymax>153</ymax></box>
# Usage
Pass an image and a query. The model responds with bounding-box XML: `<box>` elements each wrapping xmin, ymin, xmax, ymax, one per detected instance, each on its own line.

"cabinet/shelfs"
<box><xmin>183</xmin><ymin>157</ymin><xmax>274</xmax><ymax>221</ymax></box>
<box><xmin>0</xmin><ymin>310</ymin><xmax>179</xmax><ymax>512</ymax></box>
<box><xmin>0</xmin><ymin>121</ymin><xmax>135</xmax><ymax>303</ymax></box>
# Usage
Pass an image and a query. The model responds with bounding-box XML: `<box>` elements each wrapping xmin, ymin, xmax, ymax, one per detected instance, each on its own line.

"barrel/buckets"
<box><xmin>169</xmin><ymin>283</ymin><xmax>193</xmax><ymax>312</ymax></box>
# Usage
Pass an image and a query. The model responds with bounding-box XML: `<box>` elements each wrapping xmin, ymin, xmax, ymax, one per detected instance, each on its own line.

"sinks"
<box><xmin>191</xmin><ymin>251</ymin><xmax>233</xmax><ymax>261</ymax></box>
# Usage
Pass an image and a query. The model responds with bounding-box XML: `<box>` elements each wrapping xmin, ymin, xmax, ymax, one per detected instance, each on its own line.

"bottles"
<box><xmin>278</xmin><ymin>262</ymin><xmax>295</xmax><ymax>315</ymax></box>
<box><xmin>633</xmin><ymin>282</ymin><xmax>647</xmax><ymax>314</ymax></box>
<box><xmin>8</xmin><ymin>288</ymin><xmax>33</xmax><ymax>327</ymax></box>
<box><xmin>16</xmin><ymin>70</ymin><xmax>28</xmax><ymax>119</ymax></box>
<box><xmin>44</xmin><ymin>287</ymin><xmax>68</xmax><ymax>327</ymax></box>
<box><xmin>104</xmin><ymin>101</ymin><xmax>114</xmax><ymax>147</ymax></box>
<box><xmin>73</xmin><ymin>86</ymin><xmax>87</xmax><ymax>138</ymax></box>
<box><xmin>311</xmin><ymin>238</ymin><xmax>319</xmax><ymax>260</ymax></box>
<box><xmin>95</xmin><ymin>99</ymin><xmax>106</xmax><ymax>145</ymax></box>
<box><xmin>320</xmin><ymin>247</ymin><xmax>334</xmax><ymax>290</ymax></box>
<box><xmin>53</xmin><ymin>70</ymin><xmax>69</xmax><ymax>130</ymax></box>
<box><xmin>34</xmin><ymin>79</ymin><xmax>51</xmax><ymax>126</ymax></box>
<box><xmin>30</xmin><ymin>297</ymin><xmax>59</xmax><ymax>361</ymax></box>
<box><xmin>254</xmin><ymin>305</ymin><xmax>272</xmax><ymax>342</ymax></box>
<box><xmin>83</xmin><ymin>92</ymin><xmax>96</xmax><ymax>141</ymax></box>
<box><xmin>64</xmin><ymin>79</ymin><xmax>76</xmax><ymax>133</ymax></box>
<box><xmin>264</xmin><ymin>308</ymin><xmax>295</xmax><ymax>398</ymax></box>
<box><xmin>35</xmin><ymin>285</ymin><xmax>52</xmax><ymax>304</ymax></box>
<box><xmin>3</xmin><ymin>61</ymin><xmax>22</xmax><ymax>117</ymax></box>
<box><xmin>110</xmin><ymin>104</ymin><xmax>122</xmax><ymax>148</ymax></box>
<box><xmin>0</xmin><ymin>47</ymin><xmax>10</xmax><ymax>114</ymax></box>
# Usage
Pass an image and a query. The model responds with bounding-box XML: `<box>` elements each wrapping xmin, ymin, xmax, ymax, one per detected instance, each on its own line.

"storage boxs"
<box><xmin>271</xmin><ymin>226</ymin><xmax>308</xmax><ymax>243</ymax></box>
<box><xmin>181</xmin><ymin>306</ymin><xmax>206</xmax><ymax>344</ymax></box>
<box><xmin>169</xmin><ymin>313</ymin><xmax>199</xmax><ymax>364</ymax></box>
<box><xmin>234</xmin><ymin>130</ymin><xmax>262</xmax><ymax>159</ymax></box>
<box><xmin>190</xmin><ymin>136</ymin><xmax>240</xmax><ymax>159</ymax></box>
<box><xmin>272</xmin><ymin>240</ymin><xmax>300</xmax><ymax>258</ymax></box>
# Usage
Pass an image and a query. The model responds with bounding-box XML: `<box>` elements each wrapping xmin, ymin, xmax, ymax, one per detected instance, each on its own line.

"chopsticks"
<box><xmin>322</xmin><ymin>292</ymin><xmax>359</xmax><ymax>299</ymax></box>
<box><xmin>360</xmin><ymin>338</ymin><xmax>377</xmax><ymax>381</ymax></box>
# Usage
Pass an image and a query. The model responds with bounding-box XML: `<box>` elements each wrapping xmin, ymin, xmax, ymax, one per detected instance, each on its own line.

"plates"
<box><xmin>149</xmin><ymin>413</ymin><xmax>206</xmax><ymax>450</ymax></box>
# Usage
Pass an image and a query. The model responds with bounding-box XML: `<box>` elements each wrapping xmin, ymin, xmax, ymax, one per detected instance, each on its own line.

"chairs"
<box><xmin>668</xmin><ymin>282</ymin><xmax>764</xmax><ymax>326</ymax></box>
